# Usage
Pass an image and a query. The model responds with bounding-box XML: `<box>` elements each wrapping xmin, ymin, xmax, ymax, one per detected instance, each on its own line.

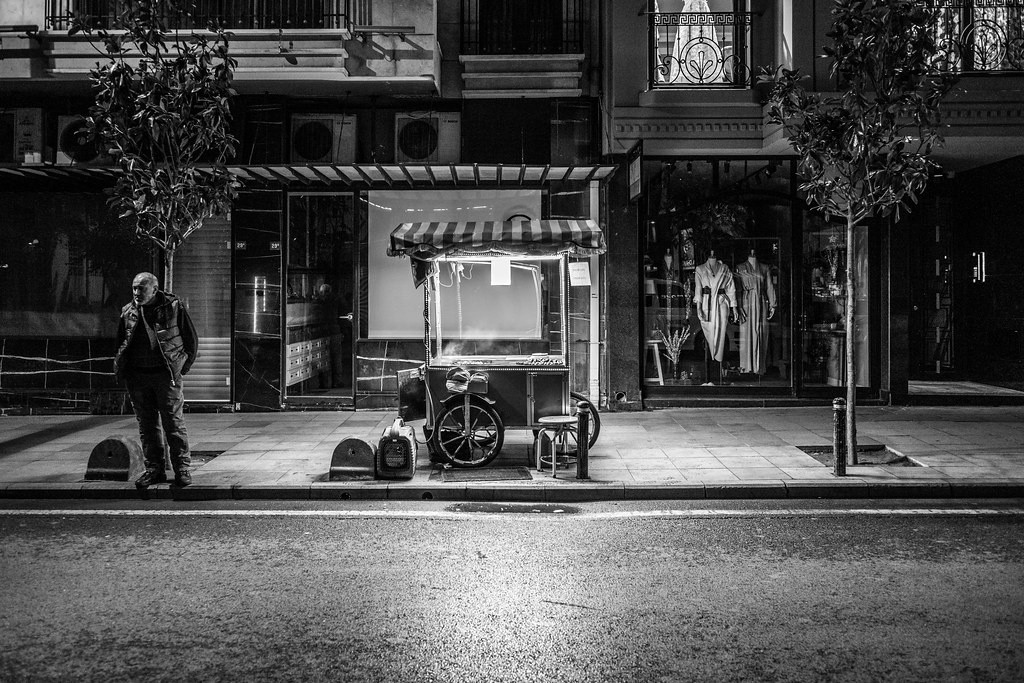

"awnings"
<box><xmin>0</xmin><ymin>161</ymin><xmax>619</xmax><ymax>189</ymax></box>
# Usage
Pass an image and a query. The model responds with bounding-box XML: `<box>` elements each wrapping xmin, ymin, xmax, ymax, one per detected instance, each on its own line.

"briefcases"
<box><xmin>375</xmin><ymin>417</ymin><xmax>416</xmax><ymax>479</ymax></box>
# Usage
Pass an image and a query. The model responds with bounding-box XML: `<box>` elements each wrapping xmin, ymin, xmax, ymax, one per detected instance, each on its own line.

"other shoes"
<box><xmin>175</xmin><ymin>470</ymin><xmax>192</xmax><ymax>484</ymax></box>
<box><xmin>135</xmin><ymin>469</ymin><xmax>167</xmax><ymax>486</ymax></box>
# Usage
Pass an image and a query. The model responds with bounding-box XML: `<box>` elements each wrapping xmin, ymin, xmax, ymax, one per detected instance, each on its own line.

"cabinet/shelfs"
<box><xmin>802</xmin><ymin>243</ymin><xmax>845</xmax><ymax>388</ymax></box>
<box><xmin>287</xmin><ymin>296</ymin><xmax>333</xmax><ymax>389</ymax></box>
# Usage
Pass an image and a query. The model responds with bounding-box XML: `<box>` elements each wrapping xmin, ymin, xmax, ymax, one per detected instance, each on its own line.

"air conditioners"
<box><xmin>289</xmin><ymin>113</ymin><xmax>356</xmax><ymax>163</ymax></box>
<box><xmin>0</xmin><ymin>107</ymin><xmax>43</xmax><ymax>162</ymax></box>
<box><xmin>56</xmin><ymin>117</ymin><xmax>114</xmax><ymax>167</ymax></box>
<box><xmin>394</xmin><ymin>111</ymin><xmax>462</xmax><ymax>165</ymax></box>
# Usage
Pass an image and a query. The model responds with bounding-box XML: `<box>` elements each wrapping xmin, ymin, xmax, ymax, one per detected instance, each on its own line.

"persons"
<box><xmin>733</xmin><ymin>255</ymin><xmax>777</xmax><ymax>374</ymax></box>
<box><xmin>693</xmin><ymin>256</ymin><xmax>738</xmax><ymax>361</ymax></box>
<box><xmin>114</xmin><ymin>272</ymin><xmax>199</xmax><ymax>487</ymax></box>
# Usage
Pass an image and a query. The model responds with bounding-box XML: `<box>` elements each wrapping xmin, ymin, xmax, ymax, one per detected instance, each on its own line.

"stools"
<box><xmin>536</xmin><ymin>415</ymin><xmax>589</xmax><ymax>478</ymax></box>
<box><xmin>644</xmin><ymin>340</ymin><xmax>664</xmax><ymax>386</ymax></box>
